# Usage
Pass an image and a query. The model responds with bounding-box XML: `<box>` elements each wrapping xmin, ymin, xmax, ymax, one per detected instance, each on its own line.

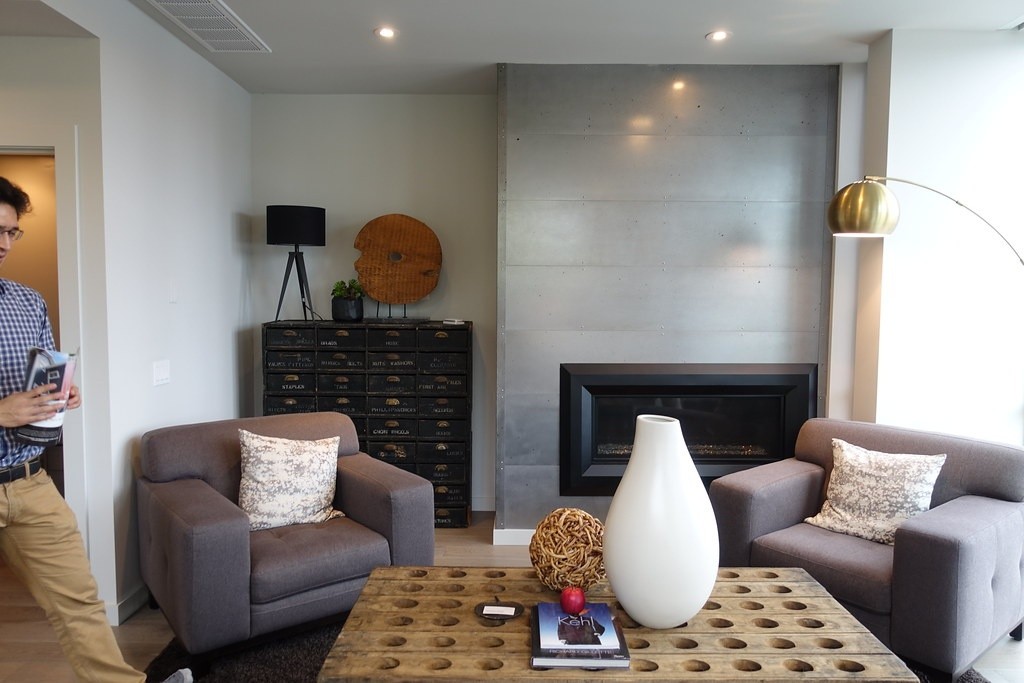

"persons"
<box><xmin>558</xmin><ymin>613</ymin><xmax>605</xmax><ymax>645</ymax></box>
<box><xmin>0</xmin><ymin>176</ymin><xmax>196</xmax><ymax>683</ymax></box>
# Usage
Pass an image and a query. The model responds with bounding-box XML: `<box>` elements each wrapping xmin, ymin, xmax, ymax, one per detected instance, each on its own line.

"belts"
<box><xmin>0</xmin><ymin>458</ymin><xmax>41</xmax><ymax>485</ymax></box>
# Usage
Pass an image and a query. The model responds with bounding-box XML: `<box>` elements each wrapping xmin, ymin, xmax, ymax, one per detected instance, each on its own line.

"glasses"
<box><xmin>0</xmin><ymin>224</ymin><xmax>24</xmax><ymax>240</ymax></box>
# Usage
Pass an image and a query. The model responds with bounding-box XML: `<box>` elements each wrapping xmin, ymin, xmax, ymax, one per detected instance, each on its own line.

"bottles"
<box><xmin>602</xmin><ymin>413</ymin><xmax>720</xmax><ymax>629</ymax></box>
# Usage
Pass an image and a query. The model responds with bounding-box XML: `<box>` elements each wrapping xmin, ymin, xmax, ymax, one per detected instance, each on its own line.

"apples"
<box><xmin>560</xmin><ymin>584</ymin><xmax>585</xmax><ymax>614</ymax></box>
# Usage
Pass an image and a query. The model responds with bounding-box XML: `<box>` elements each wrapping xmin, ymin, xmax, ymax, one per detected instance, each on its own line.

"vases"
<box><xmin>601</xmin><ymin>414</ymin><xmax>719</xmax><ymax>629</ymax></box>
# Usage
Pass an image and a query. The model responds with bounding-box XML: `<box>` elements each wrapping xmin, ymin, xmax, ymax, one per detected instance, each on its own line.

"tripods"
<box><xmin>275</xmin><ymin>245</ymin><xmax>316</xmax><ymax>324</ymax></box>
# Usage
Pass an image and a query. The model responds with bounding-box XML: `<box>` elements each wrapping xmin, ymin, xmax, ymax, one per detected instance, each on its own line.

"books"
<box><xmin>12</xmin><ymin>345</ymin><xmax>80</xmax><ymax>446</ymax></box>
<box><xmin>530</xmin><ymin>603</ymin><xmax>632</xmax><ymax>669</ymax></box>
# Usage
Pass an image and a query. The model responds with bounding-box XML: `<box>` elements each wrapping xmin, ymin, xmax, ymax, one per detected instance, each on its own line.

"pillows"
<box><xmin>237</xmin><ymin>427</ymin><xmax>345</xmax><ymax>531</ymax></box>
<box><xmin>803</xmin><ymin>438</ymin><xmax>948</xmax><ymax>547</ymax></box>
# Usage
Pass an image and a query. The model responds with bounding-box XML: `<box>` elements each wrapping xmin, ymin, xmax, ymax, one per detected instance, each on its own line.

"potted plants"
<box><xmin>330</xmin><ymin>278</ymin><xmax>365</xmax><ymax>321</ymax></box>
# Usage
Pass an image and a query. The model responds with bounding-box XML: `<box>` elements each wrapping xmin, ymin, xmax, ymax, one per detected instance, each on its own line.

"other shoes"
<box><xmin>157</xmin><ymin>668</ymin><xmax>193</xmax><ymax>683</ymax></box>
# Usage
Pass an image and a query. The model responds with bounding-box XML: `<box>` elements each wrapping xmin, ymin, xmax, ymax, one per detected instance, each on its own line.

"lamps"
<box><xmin>826</xmin><ymin>176</ymin><xmax>1024</xmax><ymax>268</ymax></box>
<box><xmin>266</xmin><ymin>204</ymin><xmax>326</xmax><ymax>321</ymax></box>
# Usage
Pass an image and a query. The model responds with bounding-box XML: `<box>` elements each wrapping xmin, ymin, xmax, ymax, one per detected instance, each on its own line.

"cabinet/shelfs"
<box><xmin>261</xmin><ymin>318</ymin><xmax>474</xmax><ymax>528</ymax></box>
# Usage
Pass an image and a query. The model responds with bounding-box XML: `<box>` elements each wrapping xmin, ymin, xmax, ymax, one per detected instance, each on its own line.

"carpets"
<box><xmin>145</xmin><ymin>593</ymin><xmax>990</xmax><ymax>683</ymax></box>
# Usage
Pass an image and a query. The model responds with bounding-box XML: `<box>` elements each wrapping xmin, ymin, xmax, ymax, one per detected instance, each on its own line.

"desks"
<box><xmin>315</xmin><ymin>561</ymin><xmax>921</xmax><ymax>683</ymax></box>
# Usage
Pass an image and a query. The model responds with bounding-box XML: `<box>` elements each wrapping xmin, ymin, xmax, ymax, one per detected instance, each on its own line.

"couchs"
<box><xmin>708</xmin><ymin>418</ymin><xmax>1024</xmax><ymax>679</ymax></box>
<box><xmin>134</xmin><ymin>411</ymin><xmax>436</xmax><ymax>651</ymax></box>
